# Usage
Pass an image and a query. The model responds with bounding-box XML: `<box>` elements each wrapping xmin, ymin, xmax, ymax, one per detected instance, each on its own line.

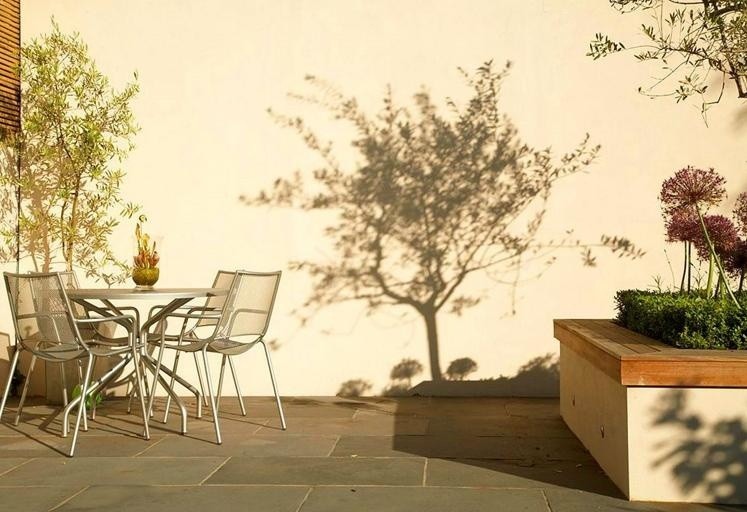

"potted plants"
<box><xmin>131</xmin><ymin>214</ymin><xmax>161</xmax><ymax>290</ymax></box>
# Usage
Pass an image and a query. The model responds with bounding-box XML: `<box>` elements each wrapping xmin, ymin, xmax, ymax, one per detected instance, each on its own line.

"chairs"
<box><xmin>1</xmin><ymin>270</ymin><xmax>287</xmax><ymax>458</ymax></box>
